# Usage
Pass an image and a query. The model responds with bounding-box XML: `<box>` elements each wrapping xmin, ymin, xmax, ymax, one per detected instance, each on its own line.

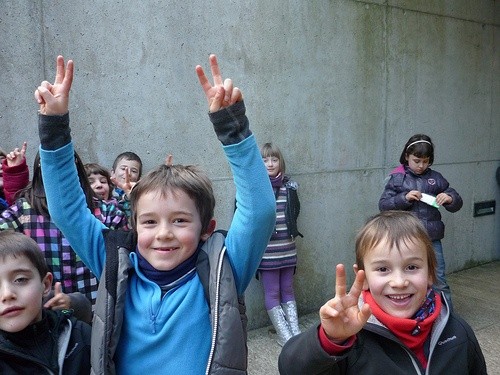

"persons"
<box><xmin>0</xmin><ymin>143</ymin><xmax>180</xmax><ymax>325</ymax></box>
<box><xmin>36</xmin><ymin>54</ymin><xmax>276</xmax><ymax>375</ymax></box>
<box><xmin>234</xmin><ymin>142</ymin><xmax>305</xmax><ymax>347</ymax></box>
<box><xmin>0</xmin><ymin>231</ymin><xmax>93</xmax><ymax>375</ymax></box>
<box><xmin>278</xmin><ymin>211</ymin><xmax>489</xmax><ymax>375</ymax></box>
<box><xmin>378</xmin><ymin>134</ymin><xmax>463</xmax><ymax>311</ymax></box>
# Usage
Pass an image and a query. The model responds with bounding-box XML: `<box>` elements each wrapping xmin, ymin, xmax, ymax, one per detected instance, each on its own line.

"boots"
<box><xmin>281</xmin><ymin>299</ymin><xmax>302</xmax><ymax>336</ymax></box>
<box><xmin>267</xmin><ymin>304</ymin><xmax>294</xmax><ymax>348</ymax></box>
